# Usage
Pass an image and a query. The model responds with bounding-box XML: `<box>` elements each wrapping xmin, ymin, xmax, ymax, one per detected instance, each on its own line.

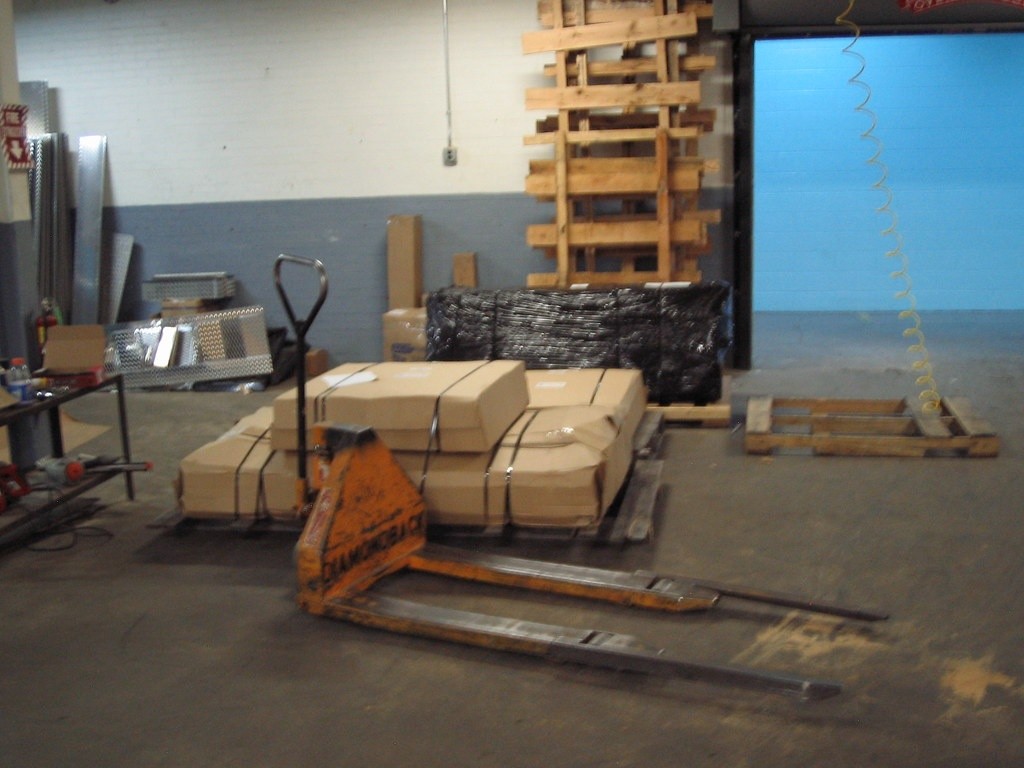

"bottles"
<box><xmin>5</xmin><ymin>356</ymin><xmax>34</xmax><ymax>406</ymax></box>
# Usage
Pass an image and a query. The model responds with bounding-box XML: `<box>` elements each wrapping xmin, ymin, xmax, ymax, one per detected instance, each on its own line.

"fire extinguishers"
<box><xmin>35</xmin><ymin>298</ymin><xmax>63</xmax><ymax>363</ymax></box>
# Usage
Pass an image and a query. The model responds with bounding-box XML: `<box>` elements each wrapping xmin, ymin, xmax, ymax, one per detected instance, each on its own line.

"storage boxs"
<box><xmin>176</xmin><ymin>214</ymin><xmax>648</xmax><ymax>531</ymax></box>
<box><xmin>160</xmin><ymin>298</ymin><xmax>217</xmax><ymax>318</ymax></box>
<box><xmin>41</xmin><ymin>326</ymin><xmax>105</xmax><ymax>373</ymax></box>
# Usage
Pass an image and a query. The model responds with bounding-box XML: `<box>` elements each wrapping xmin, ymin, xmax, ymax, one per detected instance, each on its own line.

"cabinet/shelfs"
<box><xmin>0</xmin><ymin>373</ymin><xmax>134</xmax><ymax>534</ymax></box>
